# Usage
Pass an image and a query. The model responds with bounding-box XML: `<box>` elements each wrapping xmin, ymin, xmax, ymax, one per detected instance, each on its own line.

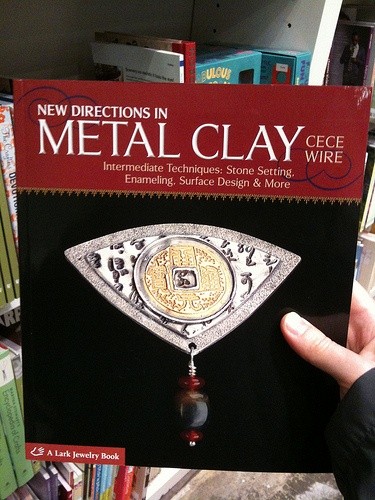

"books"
<box><xmin>0</xmin><ymin>30</ymin><xmax>375</xmax><ymax>500</ymax></box>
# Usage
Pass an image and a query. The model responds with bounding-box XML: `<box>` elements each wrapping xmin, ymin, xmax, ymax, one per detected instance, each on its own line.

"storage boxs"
<box><xmin>195</xmin><ymin>48</ymin><xmax>261</xmax><ymax>84</ymax></box>
<box><xmin>202</xmin><ymin>41</ymin><xmax>312</xmax><ymax>85</ymax></box>
